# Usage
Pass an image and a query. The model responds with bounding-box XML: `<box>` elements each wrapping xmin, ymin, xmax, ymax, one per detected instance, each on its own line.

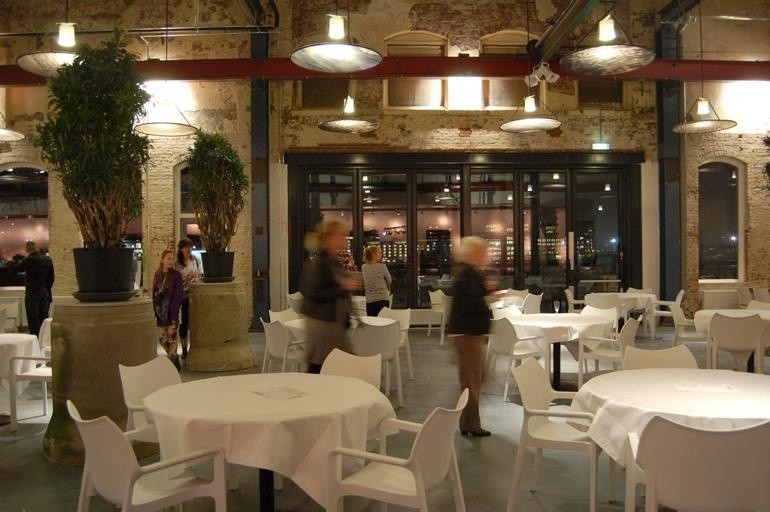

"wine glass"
<box><xmin>554</xmin><ymin>301</ymin><xmax>560</xmax><ymax>317</ymax></box>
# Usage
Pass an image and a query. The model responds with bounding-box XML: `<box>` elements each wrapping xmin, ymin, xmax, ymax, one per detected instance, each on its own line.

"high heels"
<box><xmin>462</xmin><ymin>428</ymin><xmax>492</xmax><ymax>437</ymax></box>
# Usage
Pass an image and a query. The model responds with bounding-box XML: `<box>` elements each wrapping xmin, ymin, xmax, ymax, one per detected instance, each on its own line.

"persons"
<box><xmin>152</xmin><ymin>249</ymin><xmax>183</xmax><ymax>370</ymax></box>
<box><xmin>300</xmin><ymin>220</ymin><xmax>366</xmax><ymax>373</ymax></box>
<box><xmin>445</xmin><ymin>236</ymin><xmax>503</xmax><ymax>436</ymax></box>
<box><xmin>173</xmin><ymin>237</ymin><xmax>201</xmax><ymax>359</ymax></box>
<box><xmin>361</xmin><ymin>245</ymin><xmax>392</xmax><ymax>316</ymax></box>
<box><xmin>6</xmin><ymin>242</ymin><xmax>54</xmax><ymax>336</ymax></box>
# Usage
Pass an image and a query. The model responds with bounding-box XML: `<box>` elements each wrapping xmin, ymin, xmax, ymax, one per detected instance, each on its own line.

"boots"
<box><xmin>181</xmin><ymin>336</ymin><xmax>188</xmax><ymax>359</ymax></box>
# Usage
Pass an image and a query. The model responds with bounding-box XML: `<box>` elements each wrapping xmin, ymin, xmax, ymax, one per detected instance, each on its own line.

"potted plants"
<box><xmin>179</xmin><ymin>127</ymin><xmax>251</xmax><ymax>285</ymax></box>
<box><xmin>20</xmin><ymin>21</ymin><xmax>158</xmax><ymax>305</ymax></box>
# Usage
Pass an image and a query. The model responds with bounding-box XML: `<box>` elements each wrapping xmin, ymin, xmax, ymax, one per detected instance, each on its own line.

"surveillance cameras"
<box><xmin>540</xmin><ymin>65</ymin><xmax>560</xmax><ymax>83</ymax></box>
<box><xmin>524</xmin><ymin>68</ymin><xmax>543</xmax><ymax>87</ymax></box>
